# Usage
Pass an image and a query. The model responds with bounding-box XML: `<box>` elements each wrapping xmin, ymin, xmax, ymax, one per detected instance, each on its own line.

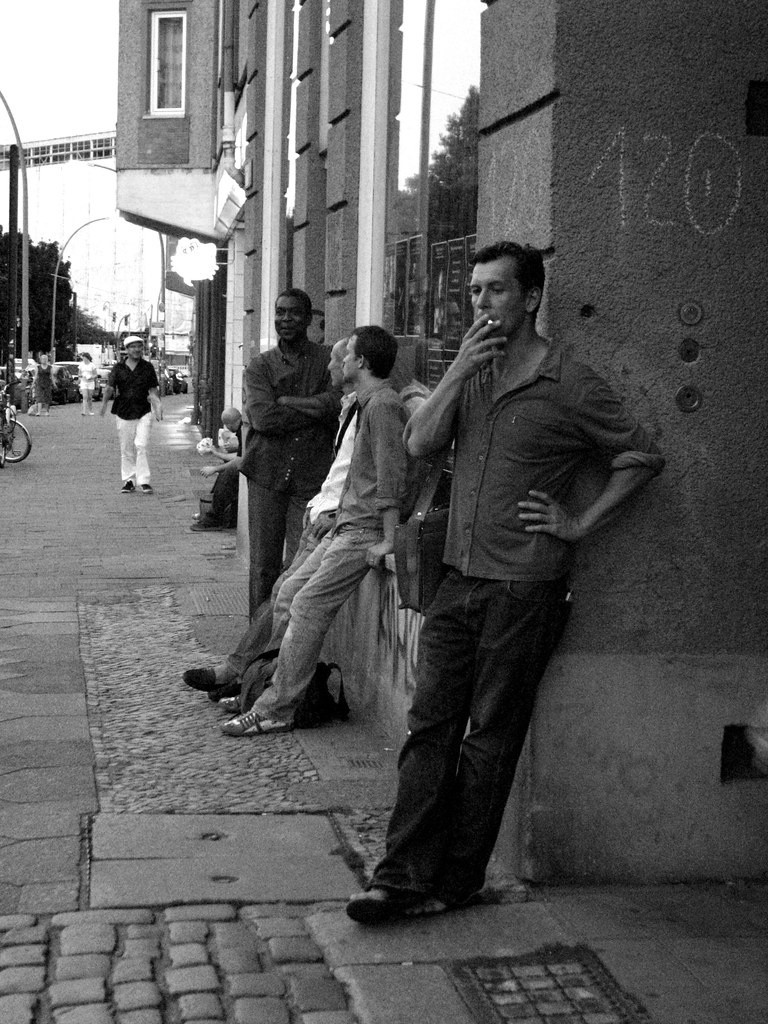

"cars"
<box><xmin>1</xmin><ymin>357</ymin><xmax>189</xmax><ymax>403</ymax></box>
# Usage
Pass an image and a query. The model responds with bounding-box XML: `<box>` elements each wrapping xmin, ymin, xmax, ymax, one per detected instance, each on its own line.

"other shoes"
<box><xmin>346</xmin><ymin>887</ymin><xmax>423</xmax><ymax>924</ymax></box>
<box><xmin>190</xmin><ymin>518</ymin><xmax>223</xmax><ymax>531</ymax></box>
<box><xmin>402</xmin><ymin>894</ymin><xmax>473</xmax><ymax>918</ymax></box>
<box><xmin>121</xmin><ymin>480</ymin><xmax>135</xmax><ymax>493</ymax></box>
<box><xmin>183</xmin><ymin>667</ymin><xmax>238</xmax><ymax>692</ymax></box>
<box><xmin>140</xmin><ymin>484</ymin><xmax>153</xmax><ymax>494</ymax></box>
<box><xmin>208</xmin><ymin>679</ymin><xmax>242</xmax><ymax>703</ymax></box>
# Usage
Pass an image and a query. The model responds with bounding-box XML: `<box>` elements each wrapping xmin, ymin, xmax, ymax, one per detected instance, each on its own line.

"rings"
<box><xmin>370</xmin><ymin>560</ymin><xmax>373</xmax><ymax>562</ymax></box>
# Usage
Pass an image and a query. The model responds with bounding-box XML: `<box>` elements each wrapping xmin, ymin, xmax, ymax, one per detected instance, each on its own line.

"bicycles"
<box><xmin>1</xmin><ymin>379</ymin><xmax>32</xmax><ymax>470</ymax></box>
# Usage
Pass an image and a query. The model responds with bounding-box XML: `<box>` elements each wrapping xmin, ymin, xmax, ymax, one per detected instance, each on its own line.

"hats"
<box><xmin>123</xmin><ymin>336</ymin><xmax>144</xmax><ymax>348</ymax></box>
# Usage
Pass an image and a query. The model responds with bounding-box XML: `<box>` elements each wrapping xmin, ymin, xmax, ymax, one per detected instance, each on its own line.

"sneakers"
<box><xmin>221</xmin><ymin>710</ymin><xmax>295</xmax><ymax>736</ymax></box>
<box><xmin>218</xmin><ymin>694</ymin><xmax>241</xmax><ymax>712</ymax></box>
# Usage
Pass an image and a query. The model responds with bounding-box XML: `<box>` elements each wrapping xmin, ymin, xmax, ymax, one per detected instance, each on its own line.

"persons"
<box><xmin>182</xmin><ymin>337</ymin><xmax>356</xmax><ymax>702</ymax></box>
<box><xmin>346</xmin><ymin>241</ymin><xmax>664</xmax><ymax>924</ymax></box>
<box><xmin>32</xmin><ymin>355</ymin><xmax>58</xmax><ymax>416</ymax></box>
<box><xmin>219</xmin><ymin>325</ymin><xmax>425</xmax><ymax>736</ymax></box>
<box><xmin>77</xmin><ymin>353</ymin><xmax>97</xmax><ymax>416</ymax></box>
<box><xmin>99</xmin><ymin>336</ymin><xmax>161</xmax><ymax>494</ymax></box>
<box><xmin>190</xmin><ymin>408</ymin><xmax>243</xmax><ymax>531</ymax></box>
<box><xmin>148</xmin><ymin>345</ymin><xmax>183</xmax><ymax>419</ymax></box>
<box><xmin>237</xmin><ymin>288</ymin><xmax>343</xmax><ymax>626</ymax></box>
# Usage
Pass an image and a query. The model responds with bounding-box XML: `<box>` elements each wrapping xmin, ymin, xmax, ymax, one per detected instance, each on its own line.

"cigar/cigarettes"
<box><xmin>488</xmin><ymin>320</ymin><xmax>492</xmax><ymax>324</ymax></box>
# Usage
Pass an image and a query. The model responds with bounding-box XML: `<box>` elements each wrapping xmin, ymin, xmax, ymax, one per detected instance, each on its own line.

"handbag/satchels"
<box><xmin>393</xmin><ymin>508</ymin><xmax>450</xmax><ymax>615</ymax></box>
<box><xmin>241</xmin><ymin>648</ymin><xmax>350</xmax><ymax>729</ymax></box>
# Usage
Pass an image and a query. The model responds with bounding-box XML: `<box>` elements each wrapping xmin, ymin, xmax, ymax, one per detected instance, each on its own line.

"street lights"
<box><xmin>50</xmin><ymin>215</ymin><xmax>111</xmax><ymax>355</ymax></box>
<box><xmin>87</xmin><ymin>161</ymin><xmax>167</xmax><ymax>306</ymax></box>
